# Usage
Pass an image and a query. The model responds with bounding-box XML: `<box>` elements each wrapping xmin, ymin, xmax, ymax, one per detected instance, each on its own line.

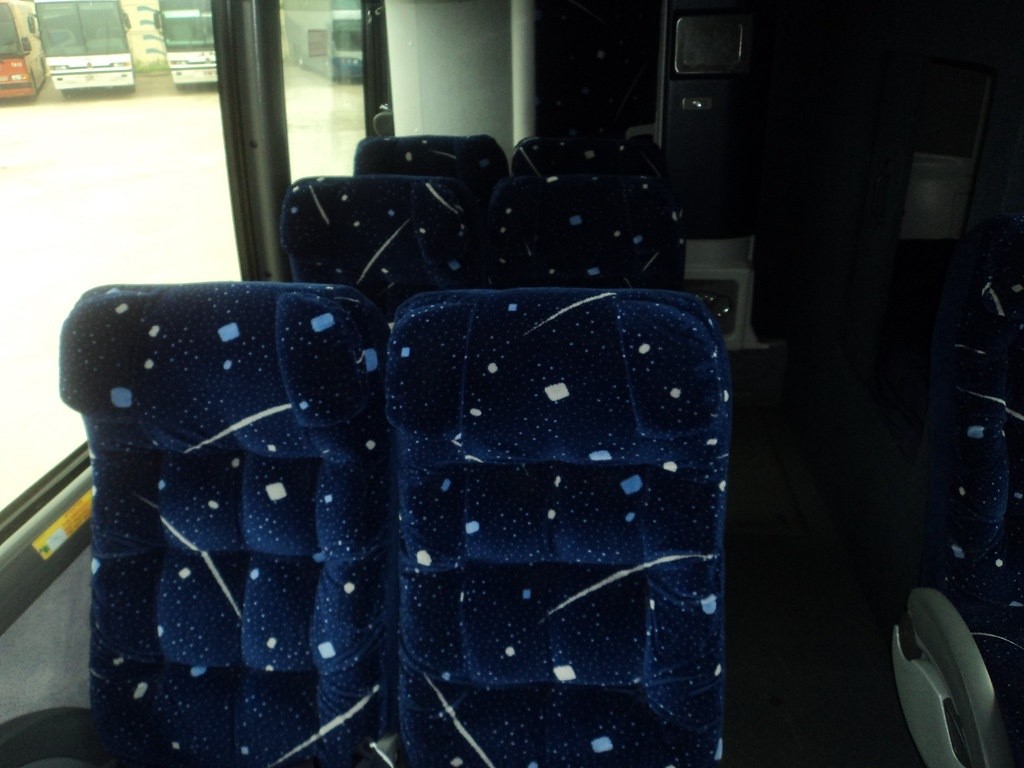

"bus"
<box><xmin>27</xmin><ymin>0</ymin><xmax>135</xmax><ymax>97</ymax></box>
<box><xmin>153</xmin><ymin>0</ymin><xmax>218</xmax><ymax>91</ymax></box>
<box><xmin>0</xmin><ymin>0</ymin><xmax>48</xmax><ymax>99</ymax></box>
<box><xmin>331</xmin><ymin>0</ymin><xmax>365</xmax><ymax>83</ymax></box>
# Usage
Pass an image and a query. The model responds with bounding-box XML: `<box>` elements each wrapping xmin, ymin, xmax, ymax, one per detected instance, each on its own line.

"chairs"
<box><xmin>0</xmin><ymin>133</ymin><xmax>1024</xmax><ymax>768</ymax></box>
